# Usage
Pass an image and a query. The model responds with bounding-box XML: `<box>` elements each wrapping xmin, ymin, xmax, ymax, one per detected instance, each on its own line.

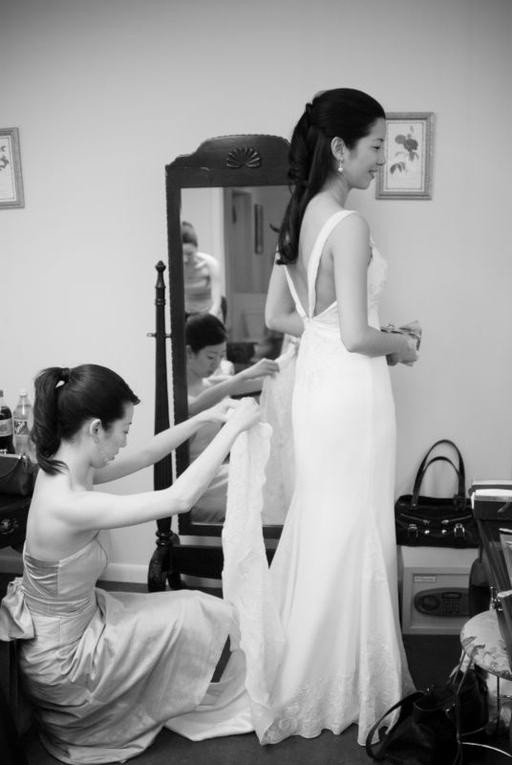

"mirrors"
<box><xmin>164</xmin><ymin>134</ymin><xmax>296</xmax><ymax>539</ymax></box>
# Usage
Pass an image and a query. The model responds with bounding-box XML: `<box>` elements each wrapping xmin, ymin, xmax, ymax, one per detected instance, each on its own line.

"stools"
<box><xmin>451</xmin><ymin>610</ymin><xmax>512</xmax><ymax>765</ymax></box>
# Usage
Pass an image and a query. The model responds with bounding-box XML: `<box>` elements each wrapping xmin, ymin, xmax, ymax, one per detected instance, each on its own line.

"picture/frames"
<box><xmin>402</xmin><ymin>567</ymin><xmax>472</xmax><ymax>636</ymax></box>
<box><xmin>376</xmin><ymin>112</ymin><xmax>435</xmax><ymax>200</ymax></box>
<box><xmin>255</xmin><ymin>205</ymin><xmax>264</xmax><ymax>254</ymax></box>
<box><xmin>0</xmin><ymin>127</ymin><xmax>25</xmax><ymax>210</ymax></box>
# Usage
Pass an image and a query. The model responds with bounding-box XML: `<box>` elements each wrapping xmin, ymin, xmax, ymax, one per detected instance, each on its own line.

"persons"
<box><xmin>184</xmin><ymin>310</ymin><xmax>280</xmax><ymax>526</ymax></box>
<box><xmin>219</xmin><ymin>88</ymin><xmax>420</xmax><ymax>746</ymax></box>
<box><xmin>0</xmin><ymin>365</ymin><xmax>261</xmax><ymax>764</ymax></box>
<box><xmin>181</xmin><ymin>221</ymin><xmax>224</xmax><ymax>323</ymax></box>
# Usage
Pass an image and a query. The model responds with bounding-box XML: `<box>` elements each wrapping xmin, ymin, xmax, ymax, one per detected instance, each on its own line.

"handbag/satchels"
<box><xmin>374</xmin><ymin>668</ymin><xmax>489</xmax><ymax>765</ymax></box>
<box><xmin>0</xmin><ymin>455</ymin><xmax>33</xmax><ymax>498</ymax></box>
<box><xmin>395</xmin><ymin>494</ymin><xmax>481</xmax><ymax>548</ymax></box>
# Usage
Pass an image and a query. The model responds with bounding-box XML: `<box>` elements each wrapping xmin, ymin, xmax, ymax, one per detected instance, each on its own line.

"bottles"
<box><xmin>13</xmin><ymin>392</ymin><xmax>33</xmax><ymax>456</ymax></box>
<box><xmin>0</xmin><ymin>389</ymin><xmax>13</xmax><ymax>454</ymax></box>
